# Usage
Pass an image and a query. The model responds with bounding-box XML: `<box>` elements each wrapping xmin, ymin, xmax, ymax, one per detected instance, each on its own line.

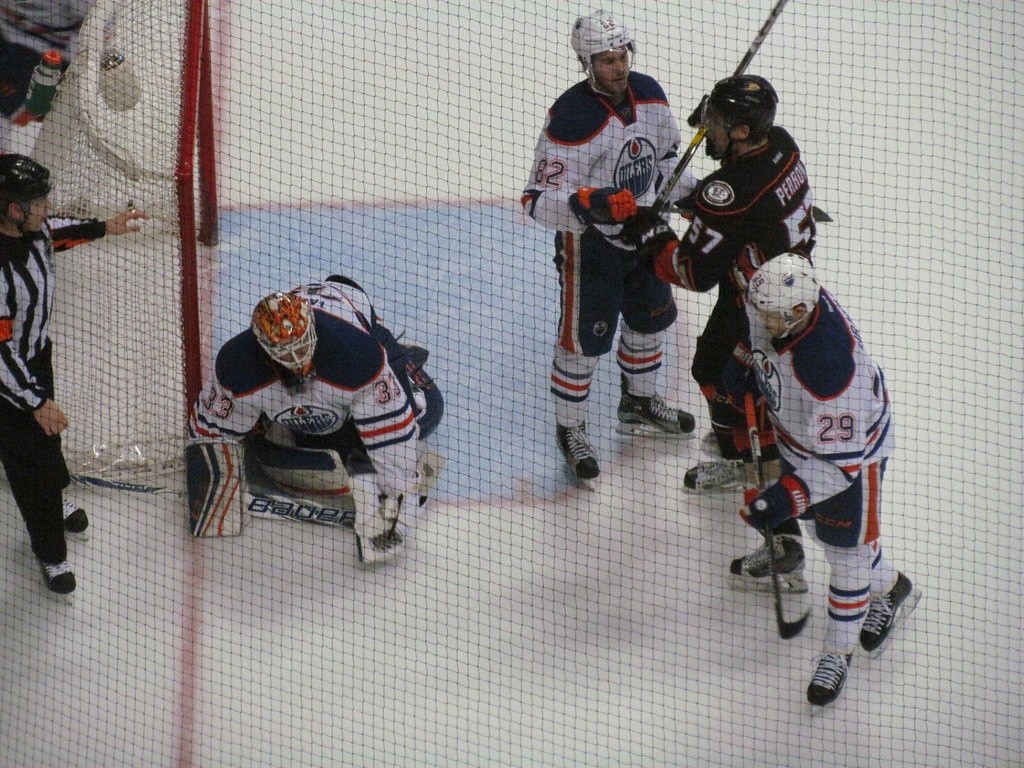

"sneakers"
<box><xmin>555</xmin><ymin>419</ymin><xmax>600</xmax><ymax>490</ymax></box>
<box><xmin>35</xmin><ymin>554</ymin><xmax>77</xmax><ymax>605</ymax></box>
<box><xmin>615</xmin><ymin>371</ymin><xmax>695</xmax><ymax>439</ymax></box>
<box><xmin>859</xmin><ymin>570</ymin><xmax>922</xmax><ymax>658</ymax></box>
<box><xmin>807</xmin><ymin>645</ymin><xmax>855</xmax><ymax>716</ymax></box>
<box><xmin>684</xmin><ymin>432</ymin><xmax>748</xmax><ymax>492</ymax></box>
<box><xmin>63</xmin><ymin>499</ymin><xmax>90</xmax><ymax>543</ymax></box>
<box><xmin>728</xmin><ymin>529</ymin><xmax>808</xmax><ymax>594</ymax></box>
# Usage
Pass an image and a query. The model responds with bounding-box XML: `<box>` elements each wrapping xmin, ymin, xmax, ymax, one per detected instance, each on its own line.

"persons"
<box><xmin>729</xmin><ymin>253</ymin><xmax>896</xmax><ymax>717</ymax></box>
<box><xmin>0</xmin><ymin>152</ymin><xmax>150</xmax><ymax>604</ymax></box>
<box><xmin>185</xmin><ymin>274</ymin><xmax>445</xmax><ymax>567</ymax></box>
<box><xmin>621</xmin><ymin>73</ymin><xmax>922</xmax><ymax>658</ymax></box>
<box><xmin>521</xmin><ymin>9</ymin><xmax>699</xmax><ymax>493</ymax></box>
<box><xmin>0</xmin><ymin>0</ymin><xmax>98</xmax><ymax>124</ymax></box>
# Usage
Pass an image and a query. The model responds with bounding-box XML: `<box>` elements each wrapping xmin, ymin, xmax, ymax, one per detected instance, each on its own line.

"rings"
<box><xmin>132</xmin><ymin>209</ymin><xmax>136</xmax><ymax>216</ymax></box>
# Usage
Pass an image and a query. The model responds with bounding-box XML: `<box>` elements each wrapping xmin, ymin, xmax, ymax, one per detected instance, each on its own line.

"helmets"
<box><xmin>707</xmin><ymin>74</ymin><xmax>779</xmax><ymax>130</ymax></box>
<box><xmin>570</xmin><ymin>9</ymin><xmax>637</xmax><ymax>67</ymax></box>
<box><xmin>252</xmin><ymin>292</ymin><xmax>316</xmax><ymax>371</ymax></box>
<box><xmin>742</xmin><ymin>250</ymin><xmax>820</xmax><ymax>329</ymax></box>
<box><xmin>0</xmin><ymin>153</ymin><xmax>51</xmax><ymax>203</ymax></box>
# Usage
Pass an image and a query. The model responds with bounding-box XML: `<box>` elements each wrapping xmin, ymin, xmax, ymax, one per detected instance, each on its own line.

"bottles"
<box><xmin>24</xmin><ymin>50</ymin><xmax>62</xmax><ymax>115</ymax></box>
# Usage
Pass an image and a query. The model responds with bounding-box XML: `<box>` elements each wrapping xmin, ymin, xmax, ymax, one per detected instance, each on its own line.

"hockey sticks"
<box><xmin>67</xmin><ymin>446</ymin><xmax>445</xmax><ymax>529</ymax></box>
<box><xmin>744</xmin><ymin>390</ymin><xmax>812</xmax><ymax>639</ymax></box>
<box><xmin>650</xmin><ymin>0</ymin><xmax>788</xmax><ymax>212</ymax></box>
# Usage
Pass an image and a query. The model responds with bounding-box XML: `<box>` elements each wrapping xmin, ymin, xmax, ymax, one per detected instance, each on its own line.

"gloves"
<box><xmin>623</xmin><ymin>205</ymin><xmax>677</xmax><ymax>265</ymax></box>
<box><xmin>738</xmin><ymin>471</ymin><xmax>811</xmax><ymax>532</ymax></box>
<box><xmin>722</xmin><ymin>340</ymin><xmax>767</xmax><ymax>414</ymax></box>
<box><xmin>687</xmin><ymin>95</ymin><xmax>720</xmax><ymax>160</ymax></box>
<box><xmin>577</xmin><ymin>185</ymin><xmax>638</xmax><ymax>222</ymax></box>
